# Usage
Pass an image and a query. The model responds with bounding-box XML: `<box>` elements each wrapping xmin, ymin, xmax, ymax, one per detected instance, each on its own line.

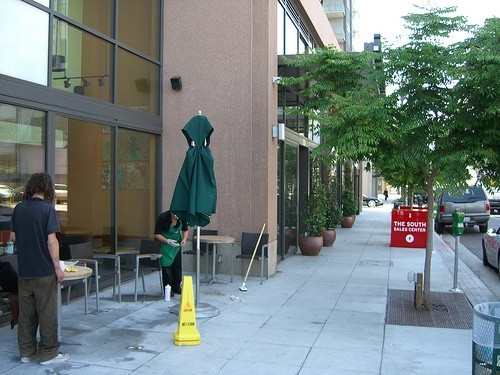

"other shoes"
<box><xmin>171</xmin><ymin>286</ymin><xmax>181</xmax><ymax>296</ymax></box>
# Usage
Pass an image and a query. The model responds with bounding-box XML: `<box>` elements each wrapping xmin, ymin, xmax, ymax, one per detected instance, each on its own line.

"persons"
<box><xmin>11</xmin><ymin>173</ymin><xmax>70</xmax><ymax>365</ymax></box>
<box><xmin>154</xmin><ymin>210</ymin><xmax>188</xmax><ymax>297</ymax></box>
<box><xmin>384</xmin><ymin>189</ymin><xmax>388</xmax><ymax>201</ymax></box>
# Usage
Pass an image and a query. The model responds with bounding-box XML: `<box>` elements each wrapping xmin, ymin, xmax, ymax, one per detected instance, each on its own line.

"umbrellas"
<box><xmin>169</xmin><ymin>113</ymin><xmax>217</xmax><ymax>307</ymax></box>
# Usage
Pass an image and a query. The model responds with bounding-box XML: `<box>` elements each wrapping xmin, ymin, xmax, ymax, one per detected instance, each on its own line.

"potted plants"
<box><xmin>340</xmin><ymin>191</ymin><xmax>356</xmax><ymax>227</ymax></box>
<box><xmin>322</xmin><ymin>201</ymin><xmax>342</xmax><ymax>247</ymax></box>
<box><xmin>299</xmin><ymin>216</ymin><xmax>323</xmax><ymax>256</ymax></box>
<box><xmin>276</xmin><ymin>209</ymin><xmax>296</xmax><ymax>255</ymax></box>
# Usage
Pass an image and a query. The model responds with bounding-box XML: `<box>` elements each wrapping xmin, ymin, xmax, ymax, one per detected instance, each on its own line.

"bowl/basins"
<box><xmin>63</xmin><ymin>261</ymin><xmax>75</xmax><ymax>271</ymax></box>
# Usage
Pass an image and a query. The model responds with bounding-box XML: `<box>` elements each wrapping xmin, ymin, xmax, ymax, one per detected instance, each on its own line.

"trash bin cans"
<box><xmin>472</xmin><ymin>302</ymin><xmax>500</xmax><ymax>375</ymax></box>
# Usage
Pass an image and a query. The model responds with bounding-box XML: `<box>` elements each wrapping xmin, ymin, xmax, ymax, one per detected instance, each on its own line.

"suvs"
<box><xmin>434</xmin><ymin>186</ymin><xmax>490</xmax><ymax>234</ymax></box>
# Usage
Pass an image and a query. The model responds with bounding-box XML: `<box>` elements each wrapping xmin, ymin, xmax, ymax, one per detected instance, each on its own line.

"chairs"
<box><xmin>182</xmin><ymin>230</ymin><xmax>218</xmax><ymax>274</ymax></box>
<box><xmin>112</xmin><ymin>239</ymin><xmax>162</xmax><ymax>298</ymax></box>
<box><xmin>236</xmin><ymin>232</ymin><xmax>269</xmax><ymax>285</ymax></box>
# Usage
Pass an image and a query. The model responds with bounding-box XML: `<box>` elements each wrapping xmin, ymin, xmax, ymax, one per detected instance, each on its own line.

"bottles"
<box><xmin>0</xmin><ymin>241</ymin><xmax>17</xmax><ymax>256</ymax></box>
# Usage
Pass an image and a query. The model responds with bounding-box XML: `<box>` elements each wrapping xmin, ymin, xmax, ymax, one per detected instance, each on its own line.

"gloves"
<box><xmin>167</xmin><ymin>239</ymin><xmax>181</xmax><ymax>247</ymax></box>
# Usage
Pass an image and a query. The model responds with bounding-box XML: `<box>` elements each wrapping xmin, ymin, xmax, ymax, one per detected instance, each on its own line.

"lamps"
<box><xmin>63</xmin><ymin>80</ymin><xmax>71</xmax><ymax>88</ymax></box>
<box><xmin>272</xmin><ymin>123</ymin><xmax>284</xmax><ymax>140</ymax></box>
<box><xmin>169</xmin><ymin>77</ymin><xmax>182</xmax><ymax>90</ymax></box>
<box><xmin>81</xmin><ymin>78</ymin><xmax>90</xmax><ymax>87</ymax></box>
<box><xmin>96</xmin><ymin>77</ymin><xmax>104</xmax><ymax>86</ymax></box>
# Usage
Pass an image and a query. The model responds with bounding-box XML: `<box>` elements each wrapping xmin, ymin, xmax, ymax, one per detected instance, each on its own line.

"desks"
<box><xmin>194</xmin><ymin>235</ymin><xmax>235</xmax><ymax>286</ymax></box>
<box><xmin>61</xmin><ymin>264</ymin><xmax>93</xmax><ymax>315</ymax></box>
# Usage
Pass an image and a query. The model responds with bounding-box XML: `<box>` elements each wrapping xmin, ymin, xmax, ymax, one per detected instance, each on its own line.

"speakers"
<box><xmin>170</xmin><ymin>76</ymin><xmax>182</xmax><ymax>90</ymax></box>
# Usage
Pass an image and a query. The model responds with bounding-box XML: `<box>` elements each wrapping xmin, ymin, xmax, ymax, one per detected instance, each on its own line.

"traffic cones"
<box><xmin>174</xmin><ymin>275</ymin><xmax>201</xmax><ymax>346</ymax></box>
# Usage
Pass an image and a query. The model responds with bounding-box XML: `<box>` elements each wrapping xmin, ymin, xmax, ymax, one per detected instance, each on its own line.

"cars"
<box><xmin>362</xmin><ymin>194</ymin><xmax>384</xmax><ymax>209</ymax></box>
<box><xmin>414</xmin><ymin>194</ymin><xmax>428</xmax><ymax>204</ymax></box>
<box><xmin>55</xmin><ymin>183</ymin><xmax>68</xmax><ymax>204</ymax></box>
<box><xmin>486</xmin><ymin>192</ymin><xmax>500</xmax><ymax>215</ymax></box>
<box><xmin>481</xmin><ymin>227</ymin><xmax>500</xmax><ymax>277</ymax></box>
<box><xmin>0</xmin><ymin>204</ymin><xmax>14</xmax><ymax>232</ymax></box>
<box><xmin>15</xmin><ymin>186</ymin><xmax>26</xmax><ymax>202</ymax></box>
<box><xmin>0</xmin><ymin>184</ymin><xmax>15</xmax><ymax>203</ymax></box>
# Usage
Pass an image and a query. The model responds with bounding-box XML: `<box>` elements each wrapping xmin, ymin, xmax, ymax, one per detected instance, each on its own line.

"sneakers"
<box><xmin>21</xmin><ymin>356</ymin><xmax>33</xmax><ymax>362</ymax></box>
<box><xmin>39</xmin><ymin>352</ymin><xmax>71</xmax><ymax>365</ymax></box>
